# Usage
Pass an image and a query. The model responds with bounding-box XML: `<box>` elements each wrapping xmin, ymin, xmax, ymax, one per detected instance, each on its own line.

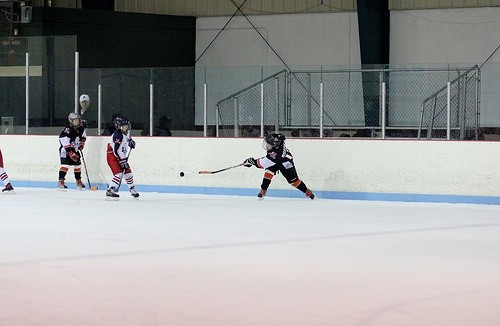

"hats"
<box><xmin>79</xmin><ymin>94</ymin><xmax>90</xmax><ymax>103</ymax></box>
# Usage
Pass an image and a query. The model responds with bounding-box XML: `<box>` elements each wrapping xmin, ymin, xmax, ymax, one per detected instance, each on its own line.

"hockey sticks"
<box><xmin>112</xmin><ymin>148</ymin><xmax>132</xmax><ymax>192</ymax></box>
<box><xmin>198</xmin><ymin>157</ymin><xmax>266</xmax><ymax>175</ymax></box>
<box><xmin>80</xmin><ymin>149</ymin><xmax>98</xmax><ymax>191</ymax></box>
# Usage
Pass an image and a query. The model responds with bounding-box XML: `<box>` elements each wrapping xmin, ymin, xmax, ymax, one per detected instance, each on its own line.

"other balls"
<box><xmin>179</xmin><ymin>171</ymin><xmax>184</xmax><ymax>177</ymax></box>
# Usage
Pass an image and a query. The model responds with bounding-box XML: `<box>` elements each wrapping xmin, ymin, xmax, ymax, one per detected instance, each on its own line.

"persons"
<box><xmin>104</xmin><ymin>116</ymin><xmax>139</xmax><ymax>201</ymax></box>
<box><xmin>57</xmin><ymin>113</ymin><xmax>87</xmax><ymax>191</ymax></box>
<box><xmin>243</xmin><ymin>133</ymin><xmax>314</xmax><ymax>200</ymax></box>
<box><xmin>141</xmin><ymin>115</ymin><xmax>173</xmax><ymax>136</ymax></box>
<box><xmin>101</xmin><ymin>112</ymin><xmax>122</xmax><ymax>136</ymax></box>
<box><xmin>79</xmin><ymin>94</ymin><xmax>97</xmax><ymax>128</ymax></box>
<box><xmin>0</xmin><ymin>150</ymin><xmax>16</xmax><ymax>194</ymax></box>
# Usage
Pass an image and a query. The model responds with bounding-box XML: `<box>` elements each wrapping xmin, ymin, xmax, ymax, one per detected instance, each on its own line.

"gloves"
<box><xmin>119</xmin><ymin>159</ymin><xmax>130</xmax><ymax>170</ymax></box>
<box><xmin>243</xmin><ymin>156</ymin><xmax>257</xmax><ymax>168</ymax></box>
<box><xmin>79</xmin><ymin>138</ymin><xmax>86</xmax><ymax>150</ymax></box>
<box><xmin>67</xmin><ymin>150</ymin><xmax>79</xmax><ymax>162</ymax></box>
<box><xmin>127</xmin><ymin>138</ymin><xmax>136</xmax><ymax>149</ymax></box>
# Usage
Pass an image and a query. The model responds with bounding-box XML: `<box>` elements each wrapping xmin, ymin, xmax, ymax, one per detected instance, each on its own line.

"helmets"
<box><xmin>113</xmin><ymin>116</ymin><xmax>131</xmax><ymax>128</ymax></box>
<box><xmin>68</xmin><ymin>112</ymin><xmax>80</xmax><ymax>122</ymax></box>
<box><xmin>261</xmin><ymin>132</ymin><xmax>281</xmax><ymax>152</ymax></box>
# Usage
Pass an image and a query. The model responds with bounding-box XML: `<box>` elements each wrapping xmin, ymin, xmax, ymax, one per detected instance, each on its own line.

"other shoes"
<box><xmin>105</xmin><ymin>189</ymin><xmax>120</xmax><ymax>201</ymax></box>
<box><xmin>129</xmin><ymin>188</ymin><xmax>140</xmax><ymax>200</ymax></box>
<box><xmin>257</xmin><ymin>190</ymin><xmax>267</xmax><ymax>201</ymax></box>
<box><xmin>76</xmin><ymin>179</ymin><xmax>86</xmax><ymax>191</ymax></box>
<box><xmin>1</xmin><ymin>182</ymin><xmax>16</xmax><ymax>195</ymax></box>
<box><xmin>306</xmin><ymin>191</ymin><xmax>316</xmax><ymax>200</ymax></box>
<box><xmin>57</xmin><ymin>180</ymin><xmax>68</xmax><ymax>192</ymax></box>
